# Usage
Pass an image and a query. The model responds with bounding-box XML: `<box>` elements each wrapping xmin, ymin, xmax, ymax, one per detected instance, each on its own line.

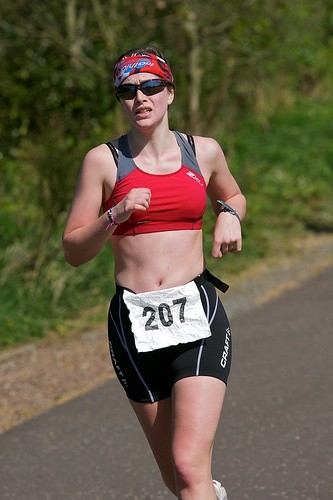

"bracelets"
<box><xmin>105</xmin><ymin>208</ymin><xmax>119</xmax><ymax>230</ymax></box>
<box><xmin>215</xmin><ymin>200</ymin><xmax>242</xmax><ymax>223</ymax></box>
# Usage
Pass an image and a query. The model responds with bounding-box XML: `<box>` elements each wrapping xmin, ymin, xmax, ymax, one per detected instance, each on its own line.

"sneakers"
<box><xmin>213</xmin><ymin>479</ymin><xmax>227</xmax><ymax>500</ymax></box>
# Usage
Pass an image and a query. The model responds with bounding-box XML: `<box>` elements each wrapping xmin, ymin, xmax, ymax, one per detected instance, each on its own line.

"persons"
<box><xmin>63</xmin><ymin>44</ymin><xmax>247</xmax><ymax>500</ymax></box>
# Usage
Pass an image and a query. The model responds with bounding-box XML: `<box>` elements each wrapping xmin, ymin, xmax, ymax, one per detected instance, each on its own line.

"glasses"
<box><xmin>116</xmin><ymin>79</ymin><xmax>169</xmax><ymax>100</ymax></box>
